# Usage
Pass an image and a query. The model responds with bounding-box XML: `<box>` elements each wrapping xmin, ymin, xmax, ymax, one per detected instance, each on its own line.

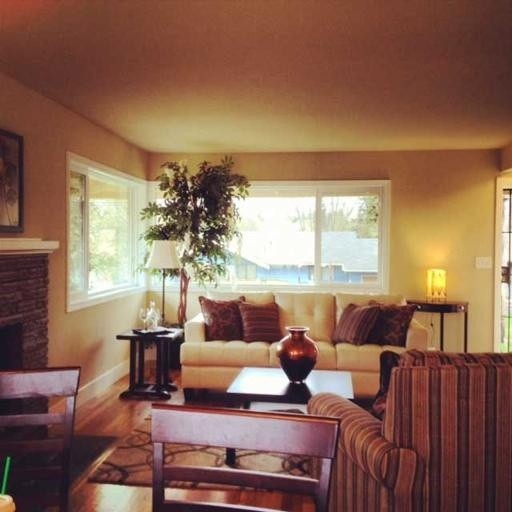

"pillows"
<box><xmin>237</xmin><ymin>301</ymin><xmax>284</xmax><ymax>343</ymax></box>
<box><xmin>198</xmin><ymin>295</ymin><xmax>245</xmax><ymax>341</ymax></box>
<box><xmin>330</xmin><ymin>303</ymin><xmax>381</xmax><ymax>346</ymax></box>
<box><xmin>368</xmin><ymin>299</ymin><xmax>417</xmax><ymax>347</ymax></box>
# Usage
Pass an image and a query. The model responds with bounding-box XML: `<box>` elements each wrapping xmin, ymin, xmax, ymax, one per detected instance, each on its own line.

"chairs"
<box><xmin>0</xmin><ymin>365</ymin><xmax>81</xmax><ymax>512</ymax></box>
<box><xmin>306</xmin><ymin>349</ymin><xmax>512</xmax><ymax>512</ymax></box>
<box><xmin>150</xmin><ymin>402</ymin><xmax>342</xmax><ymax>512</ymax></box>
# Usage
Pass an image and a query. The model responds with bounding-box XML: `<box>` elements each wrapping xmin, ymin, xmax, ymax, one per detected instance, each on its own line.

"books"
<box><xmin>132</xmin><ymin>327</ymin><xmax>175</xmax><ymax>336</ymax></box>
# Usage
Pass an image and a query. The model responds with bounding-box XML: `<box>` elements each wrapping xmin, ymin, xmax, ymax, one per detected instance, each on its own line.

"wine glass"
<box><xmin>139</xmin><ymin>307</ymin><xmax>163</xmax><ymax>332</ymax></box>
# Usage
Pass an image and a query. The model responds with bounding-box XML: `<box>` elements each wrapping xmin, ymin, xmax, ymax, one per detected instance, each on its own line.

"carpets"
<box><xmin>87</xmin><ymin>412</ymin><xmax>312</xmax><ymax>494</ymax></box>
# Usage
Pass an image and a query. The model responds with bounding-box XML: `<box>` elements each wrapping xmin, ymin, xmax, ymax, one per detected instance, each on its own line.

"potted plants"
<box><xmin>139</xmin><ymin>153</ymin><xmax>252</xmax><ymax>370</ymax></box>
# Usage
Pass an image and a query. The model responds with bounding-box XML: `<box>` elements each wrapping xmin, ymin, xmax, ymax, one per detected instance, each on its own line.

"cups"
<box><xmin>1</xmin><ymin>494</ymin><xmax>17</xmax><ymax>512</ymax></box>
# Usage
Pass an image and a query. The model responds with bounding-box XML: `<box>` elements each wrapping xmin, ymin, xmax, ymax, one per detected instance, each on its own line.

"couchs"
<box><xmin>179</xmin><ymin>292</ymin><xmax>430</xmax><ymax>403</ymax></box>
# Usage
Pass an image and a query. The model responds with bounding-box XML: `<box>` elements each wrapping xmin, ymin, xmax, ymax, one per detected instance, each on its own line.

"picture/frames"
<box><xmin>0</xmin><ymin>128</ymin><xmax>25</xmax><ymax>234</ymax></box>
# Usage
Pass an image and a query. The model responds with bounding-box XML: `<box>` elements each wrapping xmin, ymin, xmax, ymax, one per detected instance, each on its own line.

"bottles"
<box><xmin>146</xmin><ymin>301</ymin><xmax>160</xmax><ymax>331</ymax></box>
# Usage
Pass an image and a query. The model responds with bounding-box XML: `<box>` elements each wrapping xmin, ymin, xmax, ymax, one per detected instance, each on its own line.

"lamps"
<box><xmin>426</xmin><ymin>268</ymin><xmax>449</xmax><ymax>304</ymax></box>
<box><xmin>143</xmin><ymin>241</ymin><xmax>183</xmax><ymax>327</ymax></box>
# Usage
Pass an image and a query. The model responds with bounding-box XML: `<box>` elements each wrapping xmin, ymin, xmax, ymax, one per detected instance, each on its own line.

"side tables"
<box><xmin>115</xmin><ymin>328</ymin><xmax>184</xmax><ymax>400</ymax></box>
<box><xmin>406</xmin><ymin>299</ymin><xmax>469</xmax><ymax>354</ymax></box>
